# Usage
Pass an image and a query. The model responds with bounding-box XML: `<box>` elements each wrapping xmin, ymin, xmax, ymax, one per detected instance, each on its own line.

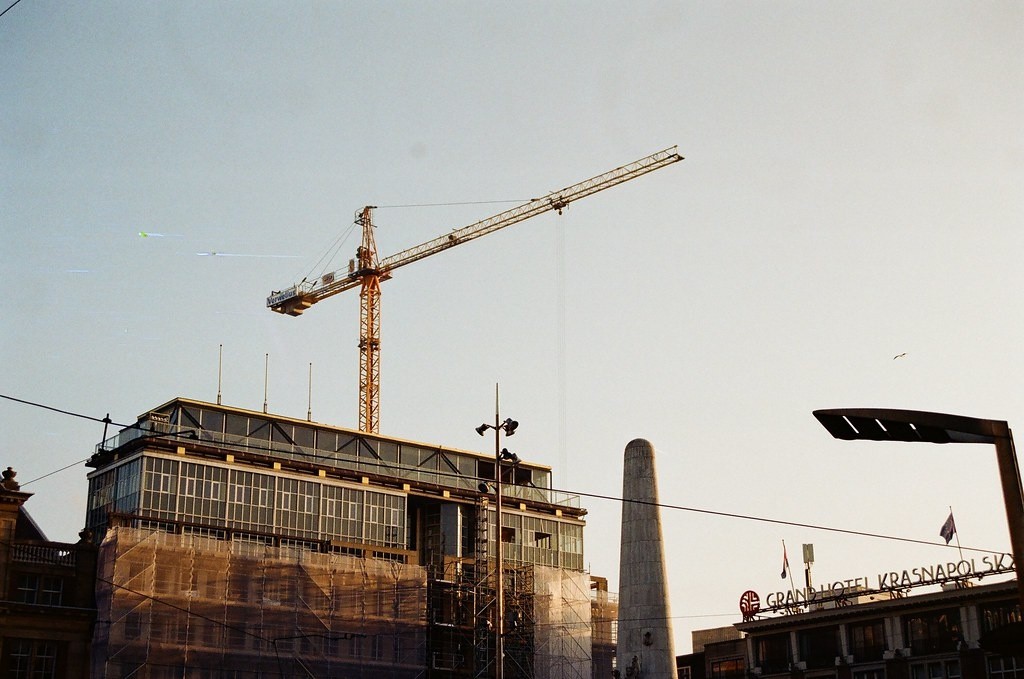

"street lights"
<box><xmin>475</xmin><ymin>383</ymin><xmax>522</xmax><ymax>679</ymax></box>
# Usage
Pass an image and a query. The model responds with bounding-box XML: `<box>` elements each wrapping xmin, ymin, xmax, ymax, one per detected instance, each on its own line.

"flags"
<box><xmin>781</xmin><ymin>542</ymin><xmax>791</xmax><ymax>580</ymax></box>
<box><xmin>938</xmin><ymin>513</ymin><xmax>955</xmax><ymax>545</ymax></box>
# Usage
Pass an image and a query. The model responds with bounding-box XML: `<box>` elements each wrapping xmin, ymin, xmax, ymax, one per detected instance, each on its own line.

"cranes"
<box><xmin>267</xmin><ymin>145</ymin><xmax>685</xmax><ymax>435</ymax></box>
